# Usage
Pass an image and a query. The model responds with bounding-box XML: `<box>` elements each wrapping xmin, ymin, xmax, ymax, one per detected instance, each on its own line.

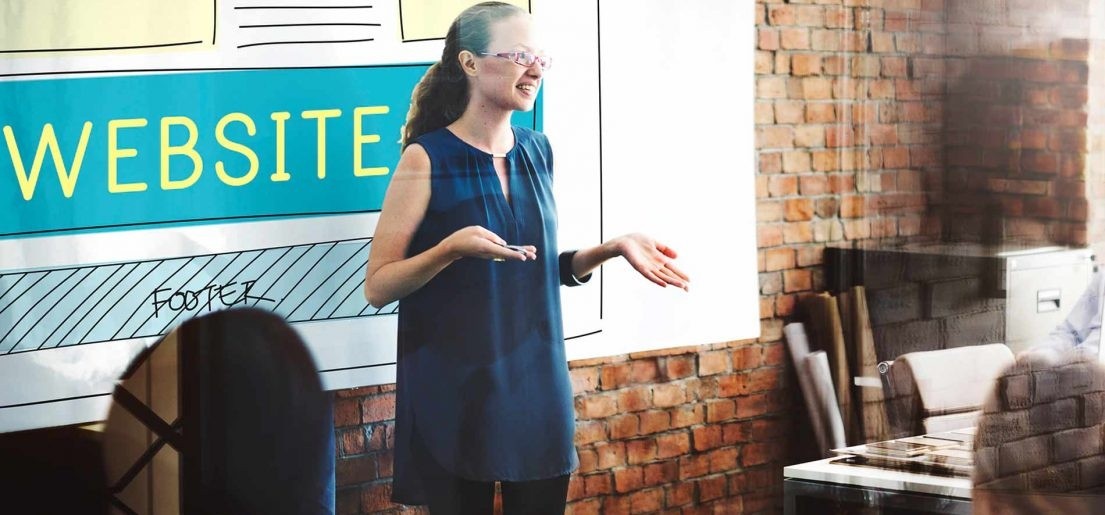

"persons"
<box><xmin>973</xmin><ymin>347</ymin><xmax>1104</xmax><ymax>514</ymax></box>
<box><xmin>363</xmin><ymin>2</ymin><xmax>692</xmax><ymax>515</ymax></box>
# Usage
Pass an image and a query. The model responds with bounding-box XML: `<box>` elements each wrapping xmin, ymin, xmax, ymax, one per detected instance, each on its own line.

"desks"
<box><xmin>784</xmin><ymin>426</ymin><xmax>979</xmax><ymax>515</ymax></box>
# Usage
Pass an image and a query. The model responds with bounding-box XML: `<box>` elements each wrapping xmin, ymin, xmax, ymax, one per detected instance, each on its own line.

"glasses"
<box><xmin>482</xmin><ymin>49</ymin><xmax>551</xmax><ymax>73</ymax></box>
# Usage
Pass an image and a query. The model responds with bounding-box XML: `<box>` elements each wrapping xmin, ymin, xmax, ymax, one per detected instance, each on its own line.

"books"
<box><xmin>866</xmin><ymin>431</ymin><xmax>975</xmax><ymax>477</ymax></box>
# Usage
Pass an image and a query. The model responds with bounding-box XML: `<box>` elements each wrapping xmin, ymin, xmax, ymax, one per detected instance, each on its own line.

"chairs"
<box><xmin>886</xmin><ymin>342</ymin><xmax>1018</xmax><ymax>434</ymax></box>
